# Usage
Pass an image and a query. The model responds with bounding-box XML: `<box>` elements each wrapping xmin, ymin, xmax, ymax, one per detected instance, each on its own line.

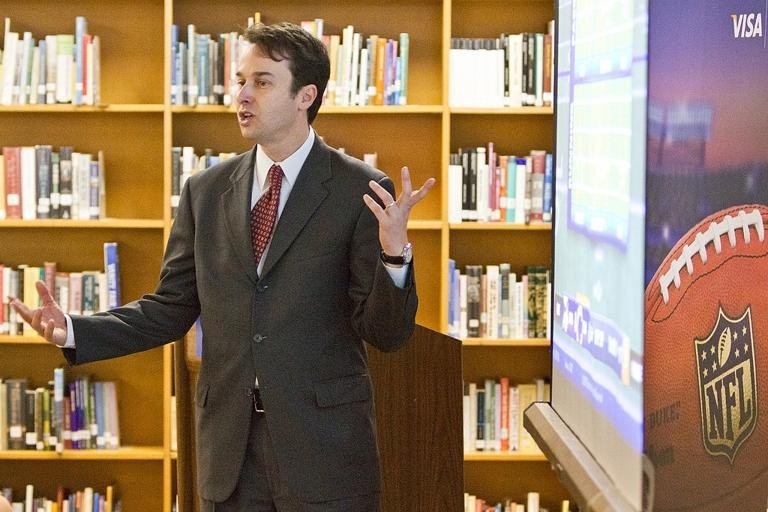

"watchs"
<box><xmin>380</xmin><ymin>243</ymin><xmax>412</xmax><ymax>265</ymax></box>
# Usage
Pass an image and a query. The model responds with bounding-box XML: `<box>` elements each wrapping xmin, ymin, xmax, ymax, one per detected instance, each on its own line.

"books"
<box><xmin>464</xmin><ymin>493</ymin><xmax>569</xmax><ymax>511</ymax></box>
<box><xmin>448</xmin><ymin>259</ymin><xmax>550</xmax><ymax>339</ymax></box>
<box><xmin>171</xmin><ymin>147</ymin><xmax>378</xmax><ymax>221</ymax></box>
<box><xmin>1</xmin><ymin>483</ymin><xmax>117</xmax><ymax>511</ymax></box>
<box><xmin>0</xmin><ymin>145</ymin><xmax>105</xmax><ymax>221</ymax></box>
<box><xmin>450</xmin><ymin>142</ymin><xmax>552</xmax><ymax>225</ymax></box>
<box><xmin>0</xmin><ymin>243</ymin><xmax>122</xmax><ymax>337</ymax></box>
<box><xmin>464</xmin><ymin>379</ymin><xmax>550</xmax><ymax>454</ymax></box>
<box><xmin>0</xmin><ymin>17</ymin><xmax>101</xmax><ymax>106</ymax></box>
<box><xmin>171</xmin><ymin>12</ymin><xmax>409</xmax><ymax>105</ymax></box>
<box><xmin>450</xmin><ymin>20</ymin><xmax>554</xmax><ymax>108</ymax></box>
<box><xmin>0</xmin><ymin>367</ymin><xmax>120</xmax><ymax>450</ymax></box>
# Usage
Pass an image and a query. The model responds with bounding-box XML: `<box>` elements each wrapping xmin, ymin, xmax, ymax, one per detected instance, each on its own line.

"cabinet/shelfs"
<box><xmin>171</xmin><ymin>2</ymin><xmax>444</xmax><ymax>336</ymax></box>
<box><xmin>0</xmin><ymin>1</ymin><xmax>172</xmax><ymax>510</ymax></box>
<box><xmin>443</xmin><ymin>1</ymin><xmax>564</xmax><ymax>511</ymax></box>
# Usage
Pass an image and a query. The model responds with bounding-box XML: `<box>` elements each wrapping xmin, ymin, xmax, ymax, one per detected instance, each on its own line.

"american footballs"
<box><xmin>645</xmin><ymin>204</ymin><xmax>766</xmax><ymax>510</ymax></box>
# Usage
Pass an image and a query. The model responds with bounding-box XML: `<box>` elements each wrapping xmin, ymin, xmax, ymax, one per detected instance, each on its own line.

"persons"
<box><xmin>6</xmin><ymin>23</ymin><xmax>438</xmax><ymax>510</ymax></box>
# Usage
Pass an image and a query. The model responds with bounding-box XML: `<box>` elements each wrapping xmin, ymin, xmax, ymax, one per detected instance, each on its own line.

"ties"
<box><xmin>250</xmin><ymin>163</ymin><xmax>285</xmax><ymax>270</ymax></box>
